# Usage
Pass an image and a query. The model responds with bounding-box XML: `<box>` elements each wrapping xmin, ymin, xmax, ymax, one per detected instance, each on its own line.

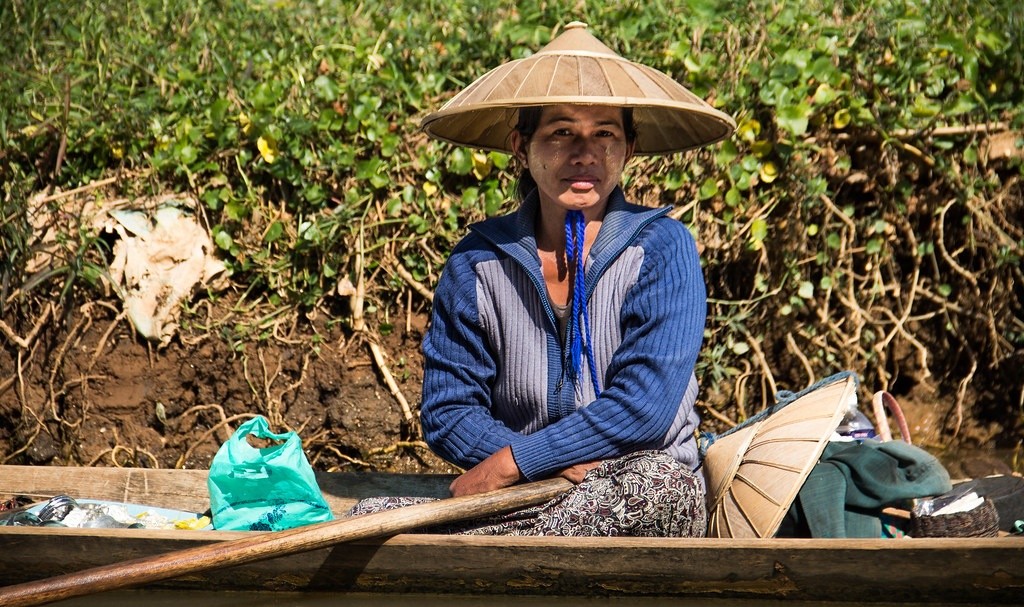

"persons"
<box><xmin>338</xmin><ymin>20</ymin><xmax>739</xmax><ymax>539</ymax></box>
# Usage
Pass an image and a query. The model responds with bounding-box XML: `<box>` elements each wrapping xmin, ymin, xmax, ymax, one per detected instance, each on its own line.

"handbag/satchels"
<box><xmin>207</xmin><ymin>416</ymin><xmax>335</xmax><ymax>531</ymax></box>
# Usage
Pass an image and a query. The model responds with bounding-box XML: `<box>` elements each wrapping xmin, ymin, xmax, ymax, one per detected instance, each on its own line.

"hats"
<box><xmin>420</xmin><ymin>20</ymin><xmax>738</xmax><ymax>157</ymax></box>
<box><xmin>699</xmin><ymin>370</ymin><xmax>859</xmax><ymax>538</ymax></box>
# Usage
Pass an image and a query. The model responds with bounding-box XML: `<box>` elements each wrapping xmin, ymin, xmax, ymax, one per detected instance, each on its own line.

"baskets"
<box><xmin>908</xmin><ymin>492</ymin><xmax>1000</xmax><ymax>537</ymax></box>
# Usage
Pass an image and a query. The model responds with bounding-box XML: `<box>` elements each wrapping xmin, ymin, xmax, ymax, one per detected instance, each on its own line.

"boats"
<box><xmin>0</xmin><ymin>463</ymin><xmax>1024</xmax><ymax>602</ymax></box>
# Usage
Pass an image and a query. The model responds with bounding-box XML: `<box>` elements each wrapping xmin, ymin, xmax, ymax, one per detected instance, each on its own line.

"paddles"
<box><xmin>0</xmin><ymin>478</ymin><xmax>572</xmax><ymax>607</ymax></box>
